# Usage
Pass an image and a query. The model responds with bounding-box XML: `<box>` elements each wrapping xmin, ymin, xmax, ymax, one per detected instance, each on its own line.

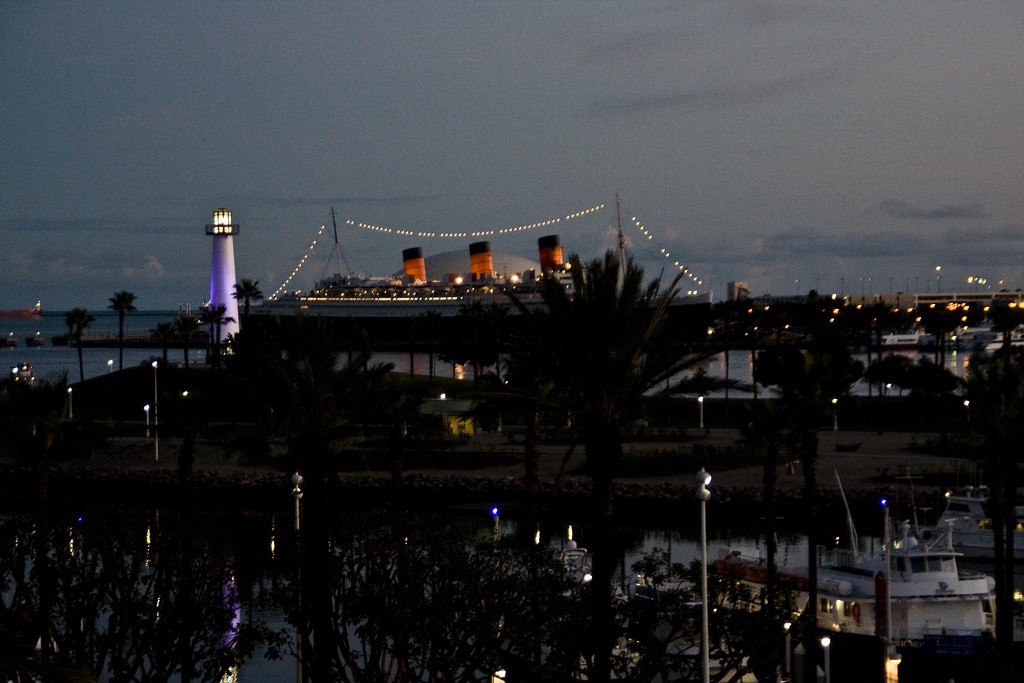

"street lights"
<box><xmin>962</xmin><ymin>399</ymin><xmax>975</xmax><ymax>435</ymax></box>
<box><xmin>831</xmin><ymin>398</ymin><xmax>839</xmax><ymax>431</ymax></box>
<box><xmin>141</xmin><ymin>404</ymin><xmax>150</xmax><ymax>430</ymax></box>
<box><xmin>151</xmin><ymin>361</ymin><xmax>162</xmax><ymax>462</ymax></box>
<box><xmin>66</xmin><ymin>386</ymin><xmax>74</xmax><ymax>418</ymax></box>
<box><xmin>290</xmin><ymin>472</ymin><xmax>304</xmax><ymax>683</ymax></box>
<box><xmin>107</xmin><ymin>358</ymin><xmax>114</xmax><ymax>372</ymax></box>
<box><xmin>696</xmin><ymin>466</ymin><xmax>714</xmax><ymax>683</ymax></box>
<box><xmin>697</xmin><ymin>396</ymin><xmax>705</xmax><ymax>429</ymax></box>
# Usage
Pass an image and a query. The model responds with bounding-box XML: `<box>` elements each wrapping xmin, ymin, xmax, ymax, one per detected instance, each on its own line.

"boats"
<box><xmin>0</xmin><ymin>330</ymin><xmax>17</xmax><ymax>347</ymax></box>
<box><xmin>25</xmin><ymin>331</ymin><xmax>44</xmax><ymax>346</ymax></box>
<box><xmin>9</xmin><ymin>362</ymin><xmax>34</xmax><ymax>385</ymax></box>
<box><xmin>535</xmin><ymin>477</ymin><xmax>1023</xmax><ymax>644</ymax></box>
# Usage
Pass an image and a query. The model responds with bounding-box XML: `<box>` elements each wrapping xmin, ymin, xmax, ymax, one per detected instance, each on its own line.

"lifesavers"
<box><xmin>852</xmin><ymin>603</ymin><xmax>861</xmax><ymax>619</ymax></box>
